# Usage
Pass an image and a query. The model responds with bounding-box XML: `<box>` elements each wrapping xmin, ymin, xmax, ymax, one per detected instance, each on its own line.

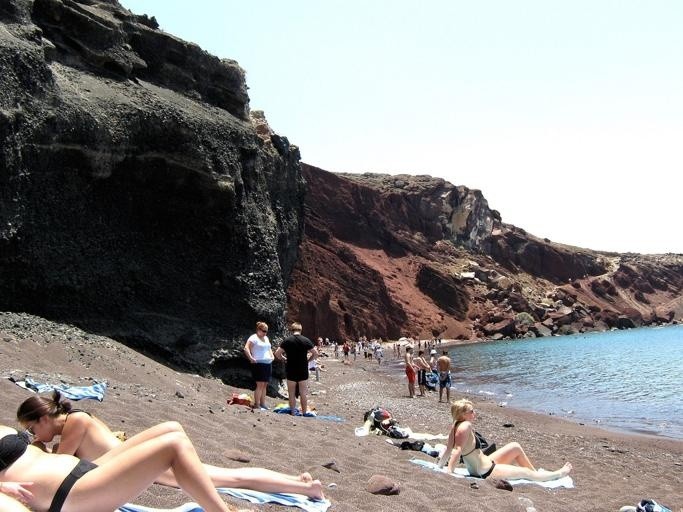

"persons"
<box><xmin>395</xmin><ymin>343</ymin><xmax>400</xmax><ymax>358</ymax></box>
<box><xmin>437</xmin><ymin>397</ymin><xmax>572</xmax><ymax>483</ymax></box>
<box><xmin>415</xmin><ymin>351</ymin><xmax>433</xmax><ymax>394</ymax></box>
<box><xmin>434</xmin><ymin>349</ymin><xmax>454</xmax><ymax>404</ymax></box>
<box><xmin>242</xmin><ymin>319</ymin><xmax>274</xmax><ymax>409</ymax></box>
<box><xmin>324</xmin><ymin>336</ymin><xmax>330</xmax><ymax>345</ymax></box>
<box><xmin>13</xmin><ymin>386</ymin><xmax>325</xmax><ymax>502</ymax></box>
<box><xmin>404</xmin><ymin>345</ymin><xmax>420</xmax><ymax>398</ymax></box>
<box><xmin>317</xmin><ymin>336</ymin><xmax>322</xmax><ymax>346</ymax></box>
<box><xmin>307</xmin><ymin>358</ymin><xmax>327</xmax><ymax>373</ymax></box>
<box><xmin>426</xmin><ymin>348</ymin><xmax>438</xmax><ymax>394</ymax></box>
<box><xmin>417</xmin><ymin>337</ymin><xmax>441</xmax><ymax>351</ymax></box>
<box><xmin>0</xmin><ymin>416</ymin><xmax>236</xmax><ymax>512</ymax></box>
<box><xmin>332</xmin><ymin>334</ymin><xmax>387</xmax><ymax>365</ymax></box>
<box><xmin>273</xmin><ymin>321</ymin><xmax>319</xmax><ymax>417</ymax></box>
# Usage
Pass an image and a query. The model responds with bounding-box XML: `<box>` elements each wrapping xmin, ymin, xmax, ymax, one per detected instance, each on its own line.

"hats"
<box><xmin>443</xmin><ymin>348</ymin><xmax>448</xmax><ymax>354</ymax></box>
<box><xmin>430</xmin><ymin>350</ymin><xmax>437</xmax><ymax>354</ymax></box>
<box><xmin>406</xmin><ymin>346</ymin><xmax>413</xmax><ymax>349</ymax></box>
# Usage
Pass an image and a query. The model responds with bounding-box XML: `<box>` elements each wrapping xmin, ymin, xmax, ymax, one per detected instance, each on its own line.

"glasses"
<box><xmin>465</xmin><ymin>410</ymin><xmax>474</xmax><ymax>414</ymax></box>
<box><xmin>25</xmin><ymin>420</ymin><xmax>39</xmax><ymax>436</ymax></box>
<box><xmin>258</xmin><ymin>327</ymin><xmax>267</xmax><ymax>332</ymax></box>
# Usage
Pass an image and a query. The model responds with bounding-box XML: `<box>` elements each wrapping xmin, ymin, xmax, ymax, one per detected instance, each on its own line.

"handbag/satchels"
<box><xmin>364</xmin><ymin>407</ymin><xmax>409</xmax><ymax>439</ymax></box>
